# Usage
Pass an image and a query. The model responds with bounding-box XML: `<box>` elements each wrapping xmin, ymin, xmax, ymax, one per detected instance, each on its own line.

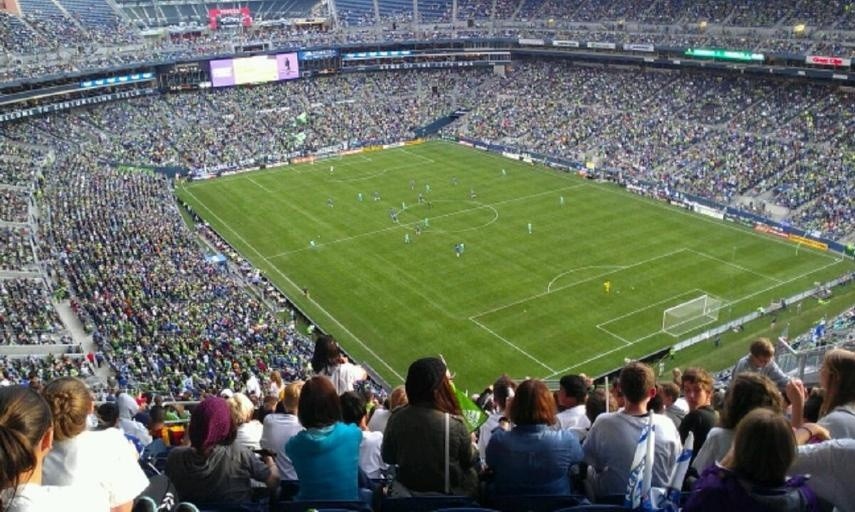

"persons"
<box><xmin>40</xmin><ymin>376</ymin><xmax>154</xmax><ymax>511</ymax></box>
<box><xmin>685</xmin><ymin>406</ymin><xmax>821</xmax><ymax>510</ymax></box>
<box><xmin>0</xmin><ymin>0</ymin><xmax>855</xmax><ymax>482</ymax></box>
<box><xmin>164</xmin><ymin>395</ymin><xmax>280</xmax><ymax>512</ymax></box>
<box><xmin>379</xmin><ymin>354</ymin><xmax>482</xmax><ymax>500</ymax></box>
<box><xmin>281</xmin><ymin>376</ymin><xmax>367</xmax><ymax>512</ymax></box>
<box><xmin>781</xmin><ymin>432</ymin><xmax>855</xmax><ymax>509</ymax></box>
<box><xmin>582</xmin><ymin>359</ymin><xmax>684</xmax><ymax>507</ymax></box>
<box><xmin>483</xmin><ymin>376</ymin><xmax>585</xmax><ymax>510</ymax></box>
<box><xmin>0</xmin><ymin>384</ymin><xmax>109</xmax><ymax>511</ymax></box>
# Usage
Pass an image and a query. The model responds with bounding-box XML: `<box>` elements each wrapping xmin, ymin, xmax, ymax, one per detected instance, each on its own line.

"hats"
<box><xmin>189</xmin><ymin>397</ymin><xmax>231</xmax><ymax>446</ymax></box>
<box><xmin>405</xmin><ymin>357</ymin><xmax>446</xmax><ymax>402</ymax></box>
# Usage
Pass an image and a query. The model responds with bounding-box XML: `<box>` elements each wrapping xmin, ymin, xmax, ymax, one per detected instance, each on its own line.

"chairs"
<box><xmin>16</xmin><ymin>1</ymin><xmax>450</xmax><ymax>28</ymax></box>
<box><xmin>96</xmin><ymin>461</ymin><xmax>838</xmax><ymax>510</ymax></box>
